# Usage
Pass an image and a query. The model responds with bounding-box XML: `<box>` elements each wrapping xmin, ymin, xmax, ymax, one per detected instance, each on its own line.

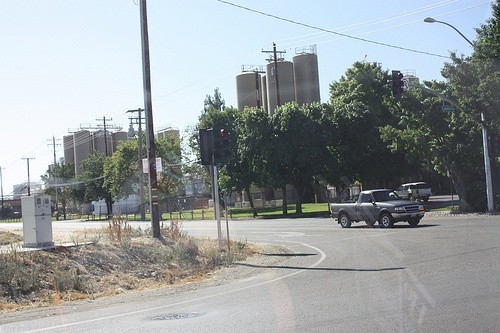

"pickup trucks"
<box><xmin>329</xmin><ymin>189</ymin><xmax>425</xmax><ymax>228</ymax></box>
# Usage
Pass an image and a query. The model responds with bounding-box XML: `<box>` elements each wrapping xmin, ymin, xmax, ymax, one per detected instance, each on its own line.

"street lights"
<box><xmin>423</xmin><ymin>17</ymin><xmax>494</xmax><ymax>211</ymax></box>
<box><xmin>391</xmin><ymin>70</ymin><xmax>403</xmax><ymax>99</ymax></box>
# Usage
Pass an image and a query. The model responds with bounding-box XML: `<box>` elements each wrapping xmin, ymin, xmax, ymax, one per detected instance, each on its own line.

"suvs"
<box><xmin>393</xmin><ymin>182</ymin><xmax>432</xmax><ymax>201</ymax></box>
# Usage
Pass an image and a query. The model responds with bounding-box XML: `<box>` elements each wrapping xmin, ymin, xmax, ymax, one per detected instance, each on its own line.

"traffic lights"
<box><xmin>210</xmin><ymin>123</ymin><xmax>230</xmax><ymax>166</ymax></box>
